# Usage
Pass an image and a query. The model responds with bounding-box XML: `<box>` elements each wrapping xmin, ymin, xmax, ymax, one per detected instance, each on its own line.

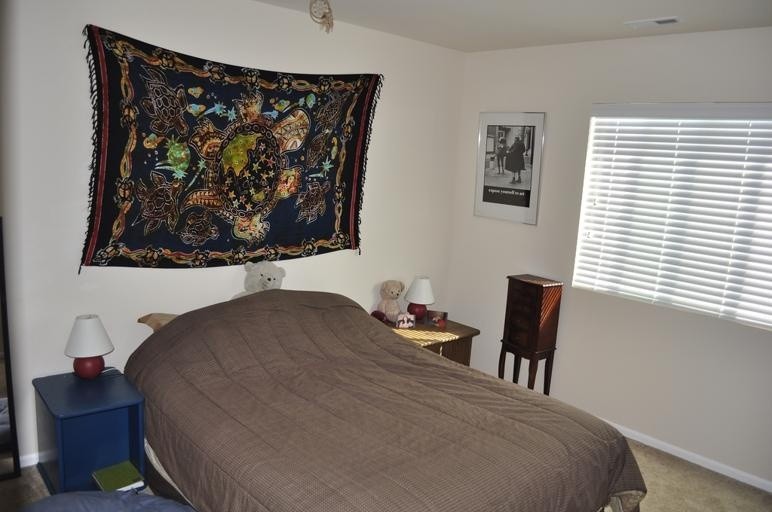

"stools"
<box><xmin>498</xmin><ymin>339</ymin><xmax>557</xmax><ymax>396</ymax></box>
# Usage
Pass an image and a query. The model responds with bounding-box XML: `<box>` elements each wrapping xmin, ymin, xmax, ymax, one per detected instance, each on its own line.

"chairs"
<box><xmin>22</xmin><ymin>490</ymin><xmax>197</xmax><ymax>512</ymax></box>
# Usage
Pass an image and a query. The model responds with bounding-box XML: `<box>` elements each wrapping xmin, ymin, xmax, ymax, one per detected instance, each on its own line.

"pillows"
<box><xmin>136</xmin><ymin>312</ymin><xmax>179</xmax><ymax>332</ymax></box>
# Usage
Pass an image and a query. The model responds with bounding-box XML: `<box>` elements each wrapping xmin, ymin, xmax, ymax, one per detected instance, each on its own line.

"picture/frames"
<box><xmin>472</xmin><ymin>110</ymin><xmax>546</xmax><ymax>226</ymax></box>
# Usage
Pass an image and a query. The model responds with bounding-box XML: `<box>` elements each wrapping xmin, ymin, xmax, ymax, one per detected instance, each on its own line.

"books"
<box><xmin>91</xmin><ymin>460</ymin><xmax>145</xmax><ymax>493</ymax></box>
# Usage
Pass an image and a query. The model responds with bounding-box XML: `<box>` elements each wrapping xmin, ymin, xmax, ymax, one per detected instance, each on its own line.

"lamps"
<box><xmin>403</xmin><ymin>276</ymin><xmax>435</xmax><ymax>324</ymax></box>
<box><xmin>63</xmin><ymin>314</ymin><xmax>116</xmax><ymax>377</ymax></box>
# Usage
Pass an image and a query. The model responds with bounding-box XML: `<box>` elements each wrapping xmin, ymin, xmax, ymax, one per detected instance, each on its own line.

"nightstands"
<box><xmin>32</xmin><ymin>366</ymin><xmax>146</xmax><ymax>496</ymax></box>
<box><xmin>386</xmin><ymin>319</ymin><xmax>480</xmax><ymax>368</ymax></box>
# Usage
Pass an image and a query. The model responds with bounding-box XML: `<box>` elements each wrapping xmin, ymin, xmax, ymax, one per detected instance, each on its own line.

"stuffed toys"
<box><xmin>378</xmin><ymin>279</ymin><xmax>406</xmax><ymax>322</ymax></box>
<box><xmin>231</xmin><ymin>260</ymin><xmax>287</xmax><ymax>299</ymax></box>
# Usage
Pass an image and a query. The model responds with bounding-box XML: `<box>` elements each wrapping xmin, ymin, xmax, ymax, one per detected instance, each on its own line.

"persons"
<box><xmin>398</xmin><ymin>317</ymin><xmax>413</xmax><ymax>329</ymax></box>
<box><xmin>495</xmin><ymin>137</ymin><xmax>505</xmax><ymax>175</ymax></box>
<box><xmin>505</xmin><ymin>136</ymin><xmax>526</xmax><ymax>185</ymax></box>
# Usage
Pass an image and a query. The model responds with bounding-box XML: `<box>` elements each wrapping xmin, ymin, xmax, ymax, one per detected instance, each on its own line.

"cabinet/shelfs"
<box><xmin>502</xmin><ymin>274</ymin><xmax>563</xmax><ymax>352</ymax></box>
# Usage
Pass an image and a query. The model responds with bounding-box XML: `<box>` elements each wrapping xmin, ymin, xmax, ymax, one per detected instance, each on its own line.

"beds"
<box><xmin>120</xmin><ymin>288</ymin><xmax>647</xmax><ymax>512</ymax></box>
